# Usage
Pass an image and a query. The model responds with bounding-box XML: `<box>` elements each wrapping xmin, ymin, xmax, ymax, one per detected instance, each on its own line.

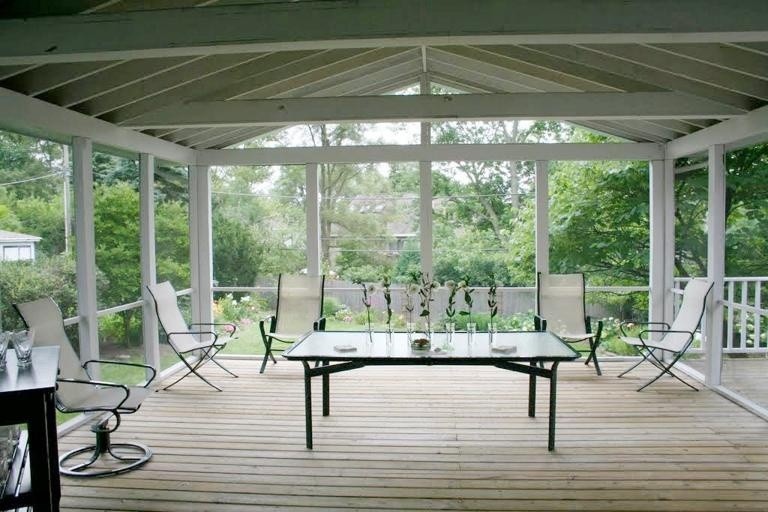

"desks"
<box><xmin>280</xmin><ymin>331</ymin><xmax>582</xmax><ymax>450</ymax></box>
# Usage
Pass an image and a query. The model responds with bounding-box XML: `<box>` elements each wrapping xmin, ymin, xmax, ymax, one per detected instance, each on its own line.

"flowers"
<box><xmin>352</xmin><ymin>273</ymin><xmax>499</xmax><ymax>348</ymax></box>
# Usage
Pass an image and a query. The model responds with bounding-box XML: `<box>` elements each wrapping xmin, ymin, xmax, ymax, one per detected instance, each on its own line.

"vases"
<box><xmin>363</xmin><ymin>322</ymin><xmax>496</xmax><ymax>348</ymax></box>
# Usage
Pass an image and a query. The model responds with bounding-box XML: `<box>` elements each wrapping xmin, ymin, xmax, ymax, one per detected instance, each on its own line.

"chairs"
<box><xmin>534</xmin><ymin>271</ymin><xmax>716</xmax><ymax>392</ymax></box>
<box><xmin>13</xmin><ymin>297</ymin><xmax>157</xmax><ymax>477</ymax></box>
<box><xmin>146</xmin><ymin>270</ymin><xmax>326</xmax><ymax>394</ymax></box>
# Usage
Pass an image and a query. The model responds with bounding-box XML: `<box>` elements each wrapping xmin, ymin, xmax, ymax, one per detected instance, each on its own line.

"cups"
<box><xmin>385</xmin><ymin>324</ymin><xmax>394</xmax><ymax>344</ymax></box>
<box><xmin>487</xmin><ymin>323</ymin><xmax>497</xmax><ymax>345</ymax></box>
<box><xmin>446</xmin><ymin>323</ymin><xmax>456</xmax><ymax>344</ymax></box>
<box><xmin>365</xmin><ymin>323</ymin><xmax>375</xmax><ymax>344</ymax></box>
<box><xmin>0</xmin><ymin>329</ymin><xmax>35</xmax><ymax>368</ymax></box>
<box><xmin>466</xmin><ymin>323</ymin><xmax>477</xmax><ymax>345</ymax></box>
<box><xmin>425</xmin><ymin>323</ymin><xmax>433</xmax><ymax>342</ymax></box>
<box><xmin>407</xmin><ymin>323</ymin><xmax>416</xmax><ymax>343</ymax></box>
